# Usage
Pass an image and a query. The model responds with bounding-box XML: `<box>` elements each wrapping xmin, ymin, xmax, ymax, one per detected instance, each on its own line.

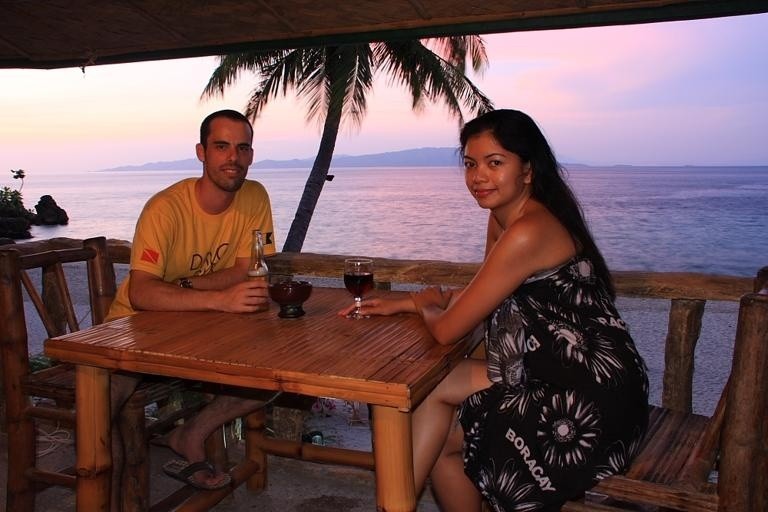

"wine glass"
<box><xmin>343</xmin><ymin>258</ymin><xmax>372</xmax><ymax>320</ymax></box>
<box><xmin>267</xmin><ymin>281</ymin><xmax>313</xmax><ymax>318</ymax></box>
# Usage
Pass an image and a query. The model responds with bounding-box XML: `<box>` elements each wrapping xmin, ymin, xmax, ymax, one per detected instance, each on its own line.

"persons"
<box><xmin>111</xmin><ymin>109</ymin><xmax>276</xmax><ymax>511</ymax></box>
<box><xmin>339</xmin><ymin>110</ymin><xmax>650</xmax><ymax>511</ymax></box>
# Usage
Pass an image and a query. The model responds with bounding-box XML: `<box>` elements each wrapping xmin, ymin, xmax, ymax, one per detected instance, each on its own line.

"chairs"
<box><xmin>1</xmin><ymin>237</ymin><xmax>238</xmax><ymax>512</ymax></box>
<box><xmin>562</xmin><ymin>263</ymin><xmax>767</xmax><ymax>511</ymax></box>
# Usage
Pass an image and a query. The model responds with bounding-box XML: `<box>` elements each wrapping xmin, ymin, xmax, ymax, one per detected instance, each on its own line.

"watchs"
<box><xmin>179</xmin><ymin>276</ymin><xmax>193</xmax><ymax>289</ymax></box>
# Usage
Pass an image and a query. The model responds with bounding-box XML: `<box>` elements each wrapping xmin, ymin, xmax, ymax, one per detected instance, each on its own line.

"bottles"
<box><xmin>246</xmin><ymin>230</ymin><xmax>270</xmax><ymax>311</ymax></box>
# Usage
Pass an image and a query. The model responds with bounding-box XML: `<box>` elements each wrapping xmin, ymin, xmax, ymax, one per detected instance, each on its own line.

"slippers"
<box><xmin>163</xmin><ymin>455</ymin><xmax>231</xmax><ymax>490</ymax></box>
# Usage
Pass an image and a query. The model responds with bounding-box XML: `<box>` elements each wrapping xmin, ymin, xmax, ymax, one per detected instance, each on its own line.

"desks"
<box><xmin>45</xmin><ymin>287</ymin><xmax>486</xmax><ymax>512</ymax></box>
